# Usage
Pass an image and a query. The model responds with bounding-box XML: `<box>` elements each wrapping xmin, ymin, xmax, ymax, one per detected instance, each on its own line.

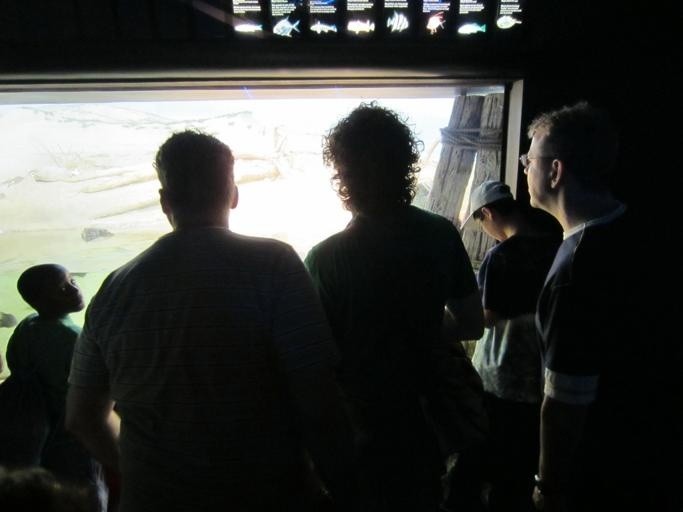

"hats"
<box><xmin>460</xmin><ymin>180</ymin><xmax>512</xmax><ymax>230</ymax></box>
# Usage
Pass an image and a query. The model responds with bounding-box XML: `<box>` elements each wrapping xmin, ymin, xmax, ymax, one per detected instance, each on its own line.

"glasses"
<box><xmin>520</xmin><ymin>154</ymin><xmax>555</xmax><ymax>168</ymax></box>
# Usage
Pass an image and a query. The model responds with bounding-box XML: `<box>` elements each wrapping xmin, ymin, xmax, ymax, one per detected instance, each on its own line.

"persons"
<box><xmin>463</xmin><ymin>176</ymin><xmax>563</xmax><ymax>429</ymax></box>
<box><xmin>64</xmin><ymin>130</ymin><xmax>340</xmax><ymax>512</ymax></box>
<box><xmin>302</xmin><ymin>105</ymin><xmax>487</xmax><ymax>511</ymax></box>
<box><xmin>519</xmin><ymin>102</ymin><xmax>682</xmax><ymax>511</ymax></box>
<box><xmin>3</xmin><ymin>464</ymin><xmax>109</xmax><ymax>512</ymax></box>
<box><xmin>1</xmin><ymin>376</ymin><xmax>117</xmax><ymax>498</ymax></box>
<box><xmin>6</xmin><ymin>260</ymin><xmax>84</xmax><ymax>413</ymax></box>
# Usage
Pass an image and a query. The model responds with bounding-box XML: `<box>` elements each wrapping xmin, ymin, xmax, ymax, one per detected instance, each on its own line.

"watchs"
<box><xmin>534</xmin><ymin>474</ymin><xmax>553</xmax><ymax>495</ymax></box>
